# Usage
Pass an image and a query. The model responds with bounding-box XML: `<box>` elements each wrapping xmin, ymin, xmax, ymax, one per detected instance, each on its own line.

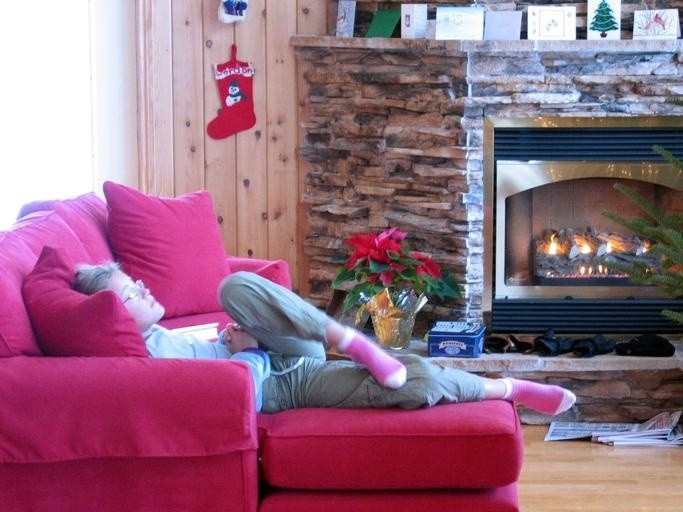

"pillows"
<box><xmin>103</xmin><ymin>182</ymin><xmax>231</xmax><ymax>317</ymax></box>
<box><xmin>1</xmin><ymin>210</ymin><xmax>95</xmax><ymax>358</ymax></box>
<box><xmin>14</xmin><ymin>191</ymin><xmax>108</xmax><ymax>265</ymax></box>
<box><xmin>21</xmin><ymin>243</ymin><xmax>150</xmax><ymax>357</ymax></box>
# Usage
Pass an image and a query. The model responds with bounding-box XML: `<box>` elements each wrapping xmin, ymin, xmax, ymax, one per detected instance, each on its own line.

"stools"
<box><xmin>259</xmin><ymin>400</ymin><xmax>524</xmax><ymax>512</ymax></box>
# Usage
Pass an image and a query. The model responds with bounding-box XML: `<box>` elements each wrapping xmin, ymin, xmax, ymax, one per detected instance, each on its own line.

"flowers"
<box><xmin>330</xmin><ymin>226</ymin><xmax>463</xmax><ymax>324</ymax></box>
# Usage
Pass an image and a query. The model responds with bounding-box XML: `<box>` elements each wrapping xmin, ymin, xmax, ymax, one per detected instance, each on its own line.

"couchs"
<box><xmin>0</xmin><ymin>257</ymin><xmax>292</xmax><ymax>512</ymax></box>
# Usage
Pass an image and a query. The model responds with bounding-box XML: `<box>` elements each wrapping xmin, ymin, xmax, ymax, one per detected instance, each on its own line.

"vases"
<box><xmin>365</xmin><ymin>287</ymin><xmax>428</xmax><ymax>351</ymax></box>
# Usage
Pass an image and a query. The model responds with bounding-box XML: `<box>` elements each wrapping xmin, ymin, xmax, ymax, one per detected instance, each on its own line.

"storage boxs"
<box><xmin>429</xmin><ymin>322</ymin><xmax>487</xmax><ymax>357</ymax></box>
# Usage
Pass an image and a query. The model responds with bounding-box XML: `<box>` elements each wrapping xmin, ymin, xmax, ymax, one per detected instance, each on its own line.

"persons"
<box><xmin>74</xmin><ymin>260</ymin><xmax>578</xmax><ymax>417</ymax></box>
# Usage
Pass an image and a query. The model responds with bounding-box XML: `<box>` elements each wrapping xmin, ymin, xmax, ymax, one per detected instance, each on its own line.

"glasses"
<box><xmin>122</xmin><ymin>279</ymin><xmax>144</xmax><ymax>304</ymax></box>
